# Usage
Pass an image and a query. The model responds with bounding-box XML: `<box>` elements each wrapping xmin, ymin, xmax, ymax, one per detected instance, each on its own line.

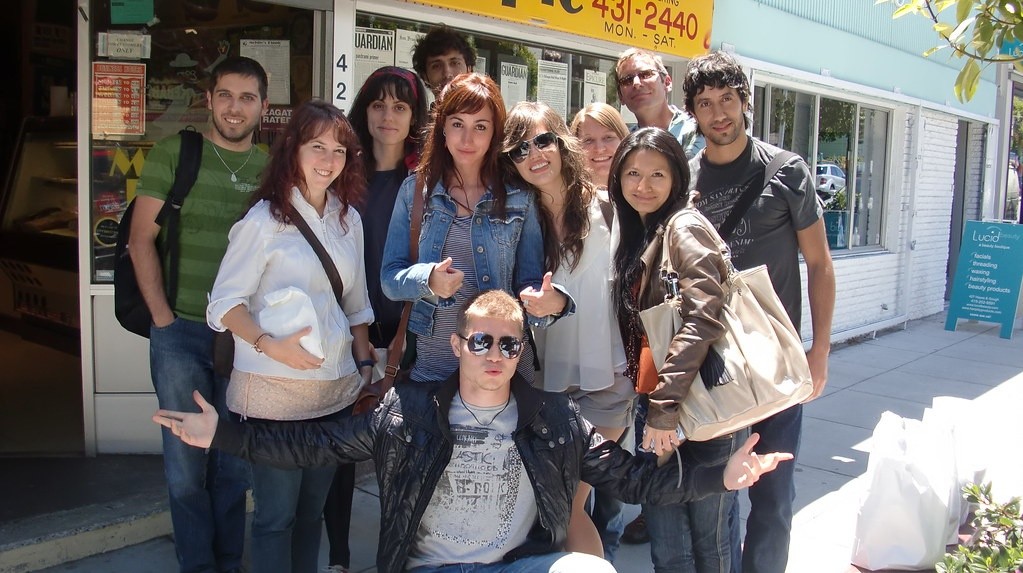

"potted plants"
<box><xmin>823</xmin><ymin>190</ymin><xmax>862</xmax><ymax>248</ymax></box>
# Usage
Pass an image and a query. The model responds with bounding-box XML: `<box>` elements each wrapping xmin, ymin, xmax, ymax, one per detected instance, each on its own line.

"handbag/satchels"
<box><xmin>113</xmin><ymin>129</ymin><xmax>203</xmax><ymax>339</ymax></box>
<box><xmin>635</xmin><ymin>208</ymin><xmax>816</xmax><ymax>441</ymax></box>
<box><xmin>350</xmin><ymin>372</ymin><xmax>397</xmax><ymax>418</ymax></box>
<box><xmin>850</xmin><ymin>396</ymin><xmax>995</xmax><ymax>571</ymax></box>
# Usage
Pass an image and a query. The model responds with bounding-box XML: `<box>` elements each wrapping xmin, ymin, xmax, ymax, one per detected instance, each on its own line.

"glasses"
<box><xmin>618</xmin><ymin>66</ymin><xmax>666</xmax><ymax>87</ymax></box>
<box><xmin>458</xmin><ymin>332</ymin><xmax>527</xmax><ymax>358</ymax></box>
<box><xmin>507</xmin><ymin>132</ymin><xmax>559</xmax><ymax>164</ymax></box>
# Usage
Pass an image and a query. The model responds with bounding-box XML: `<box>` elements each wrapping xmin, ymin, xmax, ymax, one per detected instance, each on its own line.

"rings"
<box><xmin>524</xmin><ymin>299</ymin><xmax>529</xmax><ymax>304</ymax></box>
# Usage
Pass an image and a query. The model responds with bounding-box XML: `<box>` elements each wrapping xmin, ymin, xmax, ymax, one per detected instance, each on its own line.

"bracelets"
<box><xmin>357</xmin><ymin>360</ymin><xmax>374</xmax><ymax>368</ymax></box>
<box><xmin>252</xmin><ymin>332</ymin><xmax>272</xmax><ymax>353</ymax></box>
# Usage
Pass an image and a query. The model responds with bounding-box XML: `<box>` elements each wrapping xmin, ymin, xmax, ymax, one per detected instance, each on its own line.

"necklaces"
<box><xmin>209</xmin><ymin>134</ymin><xmax>253</xmax><ymax>182</ymax></box>
<box><xmin>459</xmin><ymin>390</ymin><xmax>510</xmax><ymax>426</ymax></box>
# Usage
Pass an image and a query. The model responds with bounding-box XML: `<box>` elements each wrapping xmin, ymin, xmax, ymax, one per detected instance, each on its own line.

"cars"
<box><xmin>807</xmin><ymin>163</ymin><xmax>847</xmax><ymax>197</ymax></box>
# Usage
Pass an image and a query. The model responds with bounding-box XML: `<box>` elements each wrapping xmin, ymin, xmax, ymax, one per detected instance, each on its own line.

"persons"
<box><xmin>323</xmin><ymin>66</ymin><xmax>429</xmax><ymax>573</ymax></box>
<box><xmin>503</xmin><ymin>100</ymin><xmax>638</xmax><ymax>511</ymax></box>
<box><xmin>569</xmin><ymin>101</ymin><xmax>631</xmax><ymax>561</ymax></box>
<box><xmin>409</xmin><ymin>22</ymin><xmax>477</xmax><ymax>120</ymax></box>
<box><xmin>380</xmin><ymin>69</ymin><xmax>577</xmax><ymax>383</ymax></box>
<box><xmin>129</xmin><ymin>57</ymin><xmax>275</xmax><ymax>572</ymax></box>
<box><xmin>686</xmin><ymin>49</ymin><xmax>835</xmax><ymax>573</ymax></box>
<box><xmin>206</xmin><ymin>96</ymin><xmax>375</xmax><ymax>573</ymax></box>
<box><xmin>591</xmin><ymin>48</ymin><xmax>705</xmax><ymax>542</ymax></box>
<box><xmin>152</xmin><ymin>287</ymin><xmax>618</xmax><ymax>573</ymax></box>
<box><xmin>609</xmin><ymin>126</ymin><xmax>734</xmax><ymax>573</ymax></box>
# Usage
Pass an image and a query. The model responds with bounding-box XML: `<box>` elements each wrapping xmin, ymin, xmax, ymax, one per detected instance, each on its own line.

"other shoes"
<box><xmin>623</xmin><ymin>512</ymin><xmax>651</xmax><ymax>544</ymax></box>
